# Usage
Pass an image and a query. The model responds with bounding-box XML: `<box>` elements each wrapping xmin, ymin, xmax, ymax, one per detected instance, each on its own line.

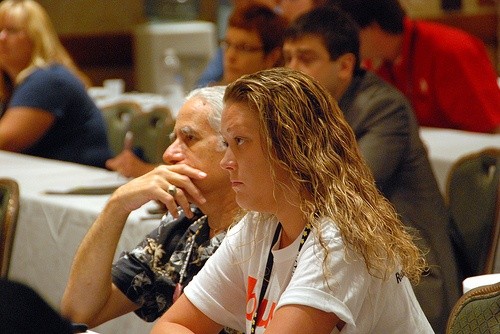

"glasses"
<box><xmin>218</xmin><ymin>38</ymin><xmax>268</xmax><ymax>56</ymax></box>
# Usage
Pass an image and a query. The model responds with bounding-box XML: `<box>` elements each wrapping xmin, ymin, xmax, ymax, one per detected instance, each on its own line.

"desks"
<box><xmin>419</xmin><ymin>125</ymin><xmax>500</xmax><ymax>199</ymax></box>
<box><xmin>0</xmin><ymin>149</ymin><xmax>156</xmax><ymax>334</ymax></box>
<box><xmin>88</xmin><ymin>86</ymin><xmax>183</xmax><ymax>120</ymax></box>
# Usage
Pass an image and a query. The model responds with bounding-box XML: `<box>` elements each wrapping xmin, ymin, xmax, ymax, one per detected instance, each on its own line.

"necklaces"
<box><xmin>173</xmin><ymin>232</ymin><xmax>196</xmax><ymax>303</ymax></box>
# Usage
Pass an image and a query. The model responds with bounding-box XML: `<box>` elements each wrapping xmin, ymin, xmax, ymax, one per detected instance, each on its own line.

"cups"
<box><xmin>104</xmin><ymin>79</ymin><xmax>123</xmax><ymax>98</ymax></box>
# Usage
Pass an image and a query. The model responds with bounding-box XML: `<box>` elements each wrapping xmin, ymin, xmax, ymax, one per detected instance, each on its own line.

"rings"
<box><xmin>167</xmin><ymin>184</ymin><xmax>177</xmax><ymax>195</ymax></box>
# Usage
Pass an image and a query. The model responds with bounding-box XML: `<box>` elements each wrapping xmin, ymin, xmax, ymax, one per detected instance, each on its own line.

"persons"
<box><xmin>59</xmin><ymin>83</ymin><xmax>250</xmax><ymax>328</ymax></box>
<box><xmin>105</xmin><ymin>0</ymin><xmax>500</xmax><ymax>334</ymax></box>
<box><xmin>0</xmin><ymin>0</ymin><xmax>113</xmax><ymax>169</ymax></box>
<box><xmin>151</xmin><ymin>67</ymin><xmax>435</xmax><ymax>334</ymax></box>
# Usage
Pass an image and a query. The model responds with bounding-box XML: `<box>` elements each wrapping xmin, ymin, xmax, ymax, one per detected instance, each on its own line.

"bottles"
<box><xmin>165</xmin><ymin>48</ymin><xmax>184</xmax><ymax>109</ymax></box>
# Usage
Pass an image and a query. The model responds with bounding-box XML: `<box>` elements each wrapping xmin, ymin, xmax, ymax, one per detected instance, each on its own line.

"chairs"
<box><xmin>444</xmin><ymin>148</ymin><xmax>500</xmax><ymax>334</ymax></box>
